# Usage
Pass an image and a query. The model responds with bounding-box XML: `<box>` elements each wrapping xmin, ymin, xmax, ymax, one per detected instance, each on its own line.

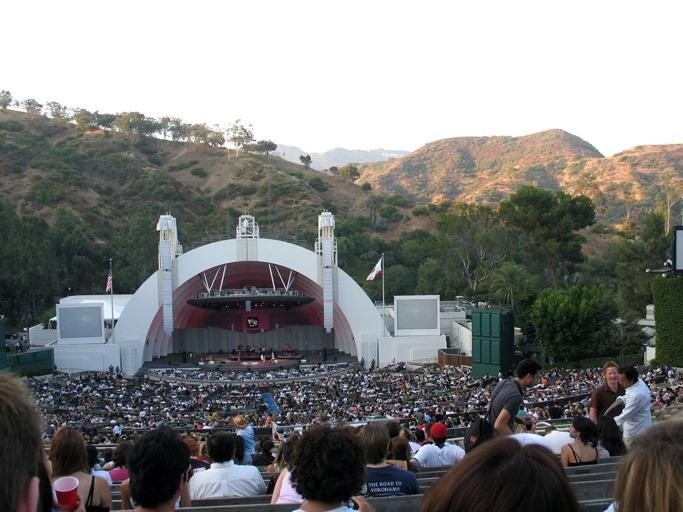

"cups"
<box><xmin>54</xmin><ymin>476</ymin><xmax>80</xmax><ymax>512</ymax></box>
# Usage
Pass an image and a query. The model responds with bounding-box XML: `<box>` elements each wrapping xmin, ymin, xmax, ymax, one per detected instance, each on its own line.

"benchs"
<box><xmin>21</xmin><ymin>365</ymin><xmax>683</xmax><ymax>512</ymax></box>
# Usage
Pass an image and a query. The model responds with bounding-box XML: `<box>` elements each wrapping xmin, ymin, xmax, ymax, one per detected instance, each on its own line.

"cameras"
<box><xmin>526</xmin><ymin>424</ymin><xmax>532</xmax><ymax>430</ymax></box>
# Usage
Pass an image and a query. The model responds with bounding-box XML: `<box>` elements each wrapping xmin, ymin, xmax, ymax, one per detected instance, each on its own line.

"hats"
<box><xmin>431</xmin><ymin>422</ymin><xmax>447</xmax><ymax>438</ymax></box>
<box><xmin>233</xmin><ymin>416</ymin><xmax>249</xmax><ymax>428</ymax></box>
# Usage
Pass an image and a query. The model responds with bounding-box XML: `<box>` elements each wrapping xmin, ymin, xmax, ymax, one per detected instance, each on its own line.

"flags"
<box><xmin>366</xmin><ymin>257</ymin><xmax>382</xmax><ymax>280</ymax></box>
<box><xmin>105</xmin><ymin>266</ymin><xmax>112</xmax><ymax>293</ymax></box>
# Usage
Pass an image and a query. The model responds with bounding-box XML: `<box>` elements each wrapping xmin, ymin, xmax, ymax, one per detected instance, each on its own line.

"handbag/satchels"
<box><xmin>463</xmin><ymin>418</ymin><xmax>504</xmax><ymax>453</ymax></box>
